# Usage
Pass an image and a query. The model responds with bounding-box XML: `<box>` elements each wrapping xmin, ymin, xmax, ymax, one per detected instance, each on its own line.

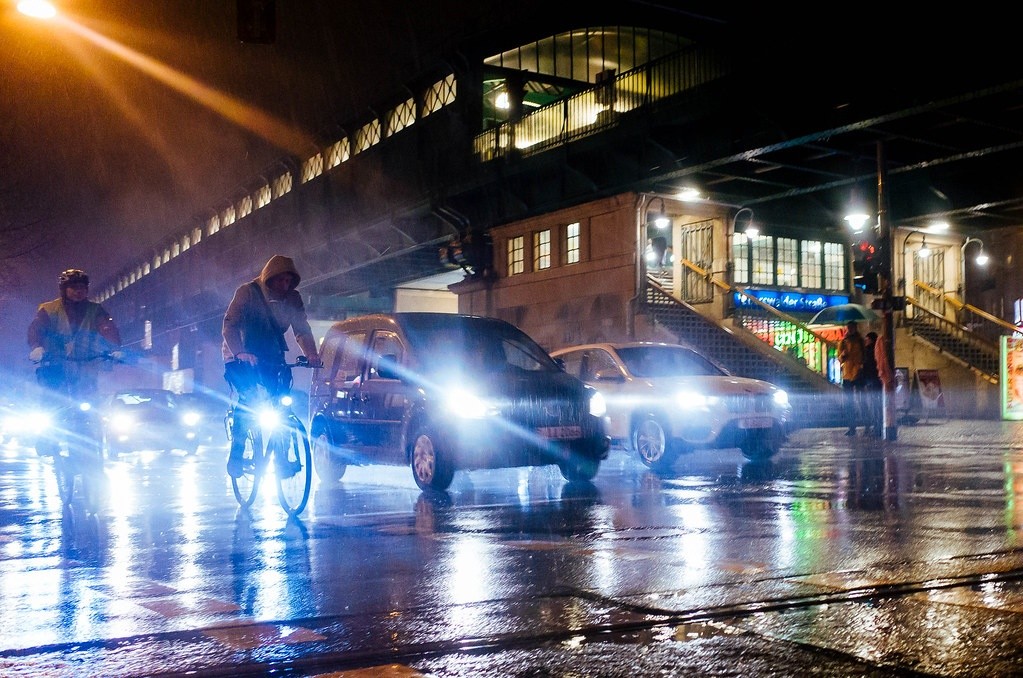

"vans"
<box><xmin>308</xmin><ymin>312</ymin><xmax>611</xmax><ymax>495</ymax></box>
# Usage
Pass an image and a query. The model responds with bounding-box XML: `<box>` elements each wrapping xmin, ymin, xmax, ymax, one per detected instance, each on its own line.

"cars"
<box><xmin>101</xmin><ymin>389</ymin><xmax>200</xmax><ymax>458</ymax></box>
<box><xmin>535</xmin><ymin>342</ymin><xmax>794</xmax><ymax>471</ymax></box>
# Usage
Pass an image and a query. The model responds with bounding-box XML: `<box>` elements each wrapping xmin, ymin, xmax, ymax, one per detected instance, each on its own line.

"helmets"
<box><xmin>57</xmin><ymin>269</ymin><xmax>89</xmax><ymax>288</ymax></box>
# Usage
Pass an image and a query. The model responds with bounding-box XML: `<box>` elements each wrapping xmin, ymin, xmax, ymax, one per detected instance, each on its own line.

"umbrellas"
<box><xmin>810</xmin><ymin>303</ymin><xmax>878</xmax><ymax>350</ymax></box>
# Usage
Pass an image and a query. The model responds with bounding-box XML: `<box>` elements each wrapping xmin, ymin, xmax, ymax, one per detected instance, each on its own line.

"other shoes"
<box><xmin>36</xmin><ymin>434</ymin><xmax>54</xmax><ymax>456</ymax></box>
<box><xmin>226</xmin><ymin>451</ymin><xmax>245</xmax><ymax>478</ymax></box>
<box><xmin>845</xmin><ymin>429</ymin><xmax>856</xmax><ymax>435</ymax></box>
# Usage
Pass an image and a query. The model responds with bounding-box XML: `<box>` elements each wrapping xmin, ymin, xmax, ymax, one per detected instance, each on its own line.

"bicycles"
<box><xmin>30</xmin><ymin>351</ymin><xmax>129</xmax><ymax>515</ymax></box>
<box><xmin>223</xmin><ymin>355</ymin><xmax>325</xmax><ymax>515</ymax></box>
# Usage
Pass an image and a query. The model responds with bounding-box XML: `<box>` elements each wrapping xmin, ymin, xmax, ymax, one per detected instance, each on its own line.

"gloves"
<box><xmin>110</xmin><ymin>351</ymin><xmax>126</xmax><ymax>361</ymax></box>
<box><xmin>29</xmin><ymin>347</ymin><xmax>45</xmax><ymax>362</ymax></box>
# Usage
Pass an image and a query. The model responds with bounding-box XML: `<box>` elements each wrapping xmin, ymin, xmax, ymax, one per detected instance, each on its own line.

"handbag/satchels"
<box><xmin>275</xmin><ymin>359</ymin><xmax>292</xmax><ymax>389</ymax></box>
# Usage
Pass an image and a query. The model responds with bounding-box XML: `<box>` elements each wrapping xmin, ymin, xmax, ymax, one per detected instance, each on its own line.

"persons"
<box><xmin>837</xmin><ymin>322</ymin><xmax>883</xmax><ymax>437</ymax></box>
<box><xmin>27</xmin><ymin>270</ymin><xmax>123</xmax><ymax>483</ymax></box>
<box><xmin>222</xmin><ymin>255</ymin><xmax>322</xmax><ymax>479</ymax></box>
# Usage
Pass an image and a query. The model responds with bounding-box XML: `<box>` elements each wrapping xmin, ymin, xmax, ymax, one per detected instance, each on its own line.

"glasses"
<box><xmin>67</xmin><ymin>284</ymin><xmax>86</xmax><ymax>289</ymax></box>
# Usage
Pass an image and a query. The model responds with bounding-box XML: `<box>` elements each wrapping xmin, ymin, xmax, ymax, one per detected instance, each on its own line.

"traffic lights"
<box><xmin>853</xmin><ymin>237</ymin><xmax>887</xmax><ymax>294</ymax></box>
